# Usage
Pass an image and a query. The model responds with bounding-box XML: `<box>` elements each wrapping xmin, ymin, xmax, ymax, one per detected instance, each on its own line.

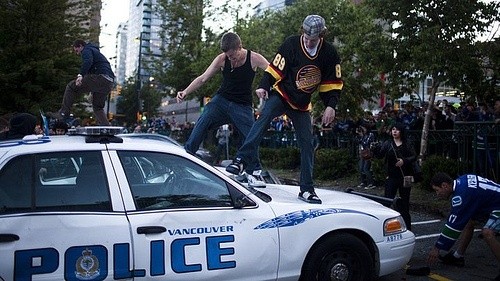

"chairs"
<box><xmin>76</xmin><ymin>157</ymin><xmax>110</xmax><ymax>211</ymax></box>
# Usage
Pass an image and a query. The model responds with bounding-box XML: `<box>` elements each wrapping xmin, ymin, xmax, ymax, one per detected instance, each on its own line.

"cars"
<box><xmin>0</xmin><ymin>126</ymin><xmax>412</xmax><ymax>281</ymax></box>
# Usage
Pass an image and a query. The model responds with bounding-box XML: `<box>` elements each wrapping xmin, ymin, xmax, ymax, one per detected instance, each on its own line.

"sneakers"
<box><xmin>226</xmin><ymin>157</ymin><xmax>244</xmax><ymax>175</ymax></box>
<box><xmin>246</xmin><ymin>170</ymin><xmax>266</xmax><ymax>188</ymax></box>
<box><xmin>439</xmin><ymin>249</ymin><xmax>464</xmax><ymax>266</ymax></box>
<box><xmin>299</xmin><ymin>191</ymin><xmax>322</xmax><ymax>204</ymax></box>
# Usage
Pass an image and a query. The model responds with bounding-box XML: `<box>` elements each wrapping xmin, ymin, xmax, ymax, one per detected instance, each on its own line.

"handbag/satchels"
<box><xmin>404</xmin><ymin>176</ymin><xmax>414</xmax><ymax>188</ymax></box>
<box><xmin>361</xmin><ymin>149</ymin><xmax>373</xmax><ymax>160</ymax></box>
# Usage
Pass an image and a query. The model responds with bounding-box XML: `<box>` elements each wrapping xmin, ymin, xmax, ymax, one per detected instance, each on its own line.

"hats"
<box><xmin>303</xmin><ymin>15</ymin><xmax>326</xmax><ymax>36</ymax></box>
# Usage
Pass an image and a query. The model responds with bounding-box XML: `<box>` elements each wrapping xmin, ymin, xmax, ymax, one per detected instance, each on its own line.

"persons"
<box><xmin>177</xmin><ymin>31</ymin><xmax>271</xmax><ymax>175</ymax></box>
<box><xmin>121</xmin><ymin>117</ymin><xmax>194</xmax><ymax>144</ymax></box>
<box><xmin>225</xmin><ymin>14</ymin><xmax>344</xmax><ymax>203</ymax></box>
<box><xmin>50</xmin><ymin>40</ymin><xmax>115</xmax><ymax>126</ymax></box>
<box><xmin>371</xmin><ymin>123</ymin><xmax>415</xmax><ymax>232</ymax></box>
<box><xmin>212</xmin><ymin>114</ymin><xmax>298</xmax><ymax>166</ymax></box>
<box><xmin>310</xmin><ymin>97</ymin><xmax>500</xmax><ymax>190</ymax></box>
<box><xmin>426</xmin><ymin>173</ymin><xmax>500</xmax><ymax>281</ymax></box>
<box><xmin>0</xmin><ymin>112</ymin><xmax>80</xmax><ymax>140</ymax></box>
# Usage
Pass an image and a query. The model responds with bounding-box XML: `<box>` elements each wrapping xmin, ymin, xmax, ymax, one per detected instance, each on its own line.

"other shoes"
<box><xmin>365</xmin><ymin>184</ymin><xmax>376</xmax><ymax>189</ymax></box>
<box><xmin>48</xmin><ymin>110</ymin><xmax>67</xmax><ymax>123</ymax></box>
<box><xmin>357</xmin><ymin>183</ymin><xmax>366</xmax><ymax>188</ymax></box>
